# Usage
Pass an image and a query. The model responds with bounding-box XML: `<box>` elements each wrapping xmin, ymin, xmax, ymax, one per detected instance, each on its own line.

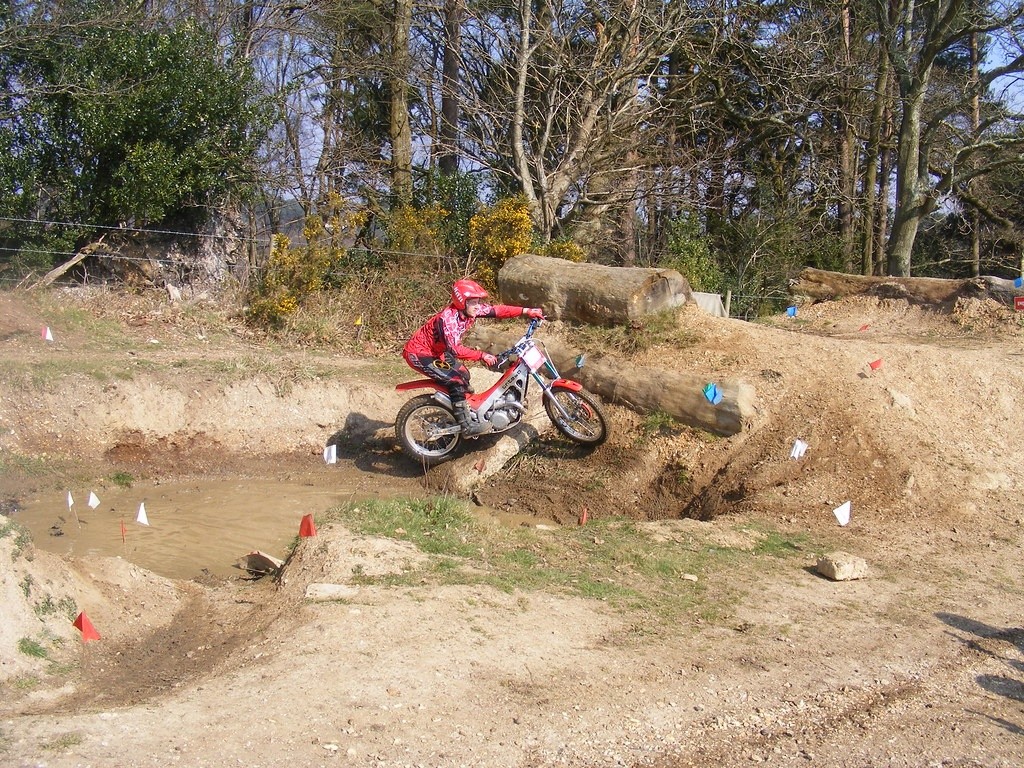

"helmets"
<box><xmin>452</xmin><ymin>279</ymin><xmax>488</xmax><ymax>310</ymax></box>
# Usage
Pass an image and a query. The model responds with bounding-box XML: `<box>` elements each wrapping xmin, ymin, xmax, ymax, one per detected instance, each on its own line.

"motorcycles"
<box><xmin>394</xmin><ymin>315</ymin><xmax>612</xmax><ymax>465</ymax></box>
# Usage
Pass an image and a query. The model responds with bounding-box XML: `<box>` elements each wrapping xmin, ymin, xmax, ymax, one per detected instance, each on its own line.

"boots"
<box><xmin>463</xmin><ymin>380</ymin><xmax>475</xmax><ymax>393</ymax></box>
<box><xmin>452</xmin><ymin>401</ymin><xmax>493</xmax><ymax>440</ymax></box>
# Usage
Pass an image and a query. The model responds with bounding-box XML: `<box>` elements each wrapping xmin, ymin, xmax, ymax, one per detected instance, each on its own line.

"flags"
<box><xmin>137</xmin><ymin>502</ymin><xmax>149</xmax><ymax>527</ymax></box>
<box><xmin>575</xmin><ymin>353</ymin><xmax>584</xmax><ymax>367</ymax></box>
<box><xmin>73</xmin><ymin>611</ymin><xmax>101</xmax><ymax>642</ymax></box>
<box><xmin>88</xmin><ymin>492</ymin><xmax>100</xmax><ymax>510</ymax></box>
<box><xmin>833</xmin><ymin>500</ymin><xmax>851</xmax><ymax>526</ymax></box>
<box><xmin>787</xmin><ymin>306</ymin><xmax>796</xmax><ymax>317</ymax></box>
<box><xmin>870</xmin><ymin>358</ymin><xmax>881</xmax><ymax>370</ymax></box>
<box><xmin>791</xmin><ymin>438</ymin><xmax>808</xmax><ymax>460</ymax></box>
<box><xmin>300</xmin><ymin>514</ymin><xmax>316</xmax><ymax>538</ymax></box>
<box><xmin>324</xmin><ymin>444</ymin><xmax>336</xmax><ymax>465</ymax></box>
<box><xmin>65</xmin><ymin>492</ymin><xmax>74</xmax><ymax>512</ymax></box>
<box><xmin>703</xmin><ymin>384</ymin><xmax>722</xmax><ymax>405</ymax></box>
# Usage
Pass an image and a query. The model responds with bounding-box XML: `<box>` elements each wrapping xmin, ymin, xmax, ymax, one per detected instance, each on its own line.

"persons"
<box><xmin>403</xmin><ymin>280</ymin><xmax>543</xmax><ymax>439</ymax></box>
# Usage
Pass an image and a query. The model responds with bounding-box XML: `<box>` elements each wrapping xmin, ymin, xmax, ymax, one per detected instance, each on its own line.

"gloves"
<box><xmin>524</xmin><ymin>308</ymin><xmax>545</xmax><ymax>320</ymax></box>
<box><xmin>480</xmin><ymin>352</ymin><xmax>498</xmax><ymax>367</ymax></box>
